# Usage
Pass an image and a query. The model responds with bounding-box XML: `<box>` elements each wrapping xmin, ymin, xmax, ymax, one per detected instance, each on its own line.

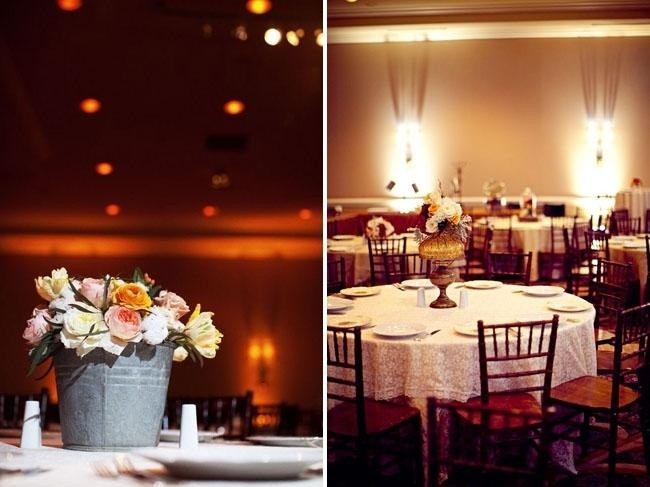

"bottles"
<box><xmin>416</xmin><ymin>289</ymin><xmax>470</xmax><ymax>309</ymax></box>
<box><xmin>20</xmin><ymin>400</ymin><xmax>198</xmax><ymax>449</ymax></box>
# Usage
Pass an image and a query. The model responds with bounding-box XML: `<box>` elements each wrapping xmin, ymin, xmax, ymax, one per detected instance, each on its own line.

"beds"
<box><xmin>1</xmin><ymin>388</ymin><xmax>323</xmax><ymax>487</ymax></box>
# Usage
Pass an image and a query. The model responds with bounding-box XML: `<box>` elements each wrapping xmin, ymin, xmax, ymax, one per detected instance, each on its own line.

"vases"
<box><xmin>50</xmin><ymin>344</ymin><xmax>174</xmax><ymax>452</ymax></box>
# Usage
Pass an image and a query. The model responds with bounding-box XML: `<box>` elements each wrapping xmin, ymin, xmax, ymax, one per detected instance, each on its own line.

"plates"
<box><xmin>330</xmin><ymin>233</ymin><xmax>415</xmax><ymax>252</ymax></box>
<box><xmin>340</xmin><ymin>287</ymin><xmax>381</xmax><ymax>297</ymax></box>
<box><xmin>328</xmin><ymin>314</ymin><xmax>371</xmax><ymax>329</ymax></box>
<box><xmin>452</xmin><ymin>318</ymin><xmax>506</xmax><ymax>337</ymax></box>
<box><xmin>521</xmin><ymin>285</ymin><xmax>564</xmax><ymax>297</ymax></box>
<box><xmin>463</xmin><ymin>280</ymin><xmax>502</xmax><ymax>289</ymax></box>
<box><xmin>327</xmin><ymin>296</ymin><xmax>352</xmax><ymax>309</ymax></box>
<box><xmin>130</xmin><ymin>429</ymin><xmax>323</xmax><ymax>477</ymax></box>
<box><xmin>372</xmin><ymin>321</ymin><xmax>427</xmax><ymax>336</ymax></box>
<box><xmin>608</xmin><ymin>233</ymin><xmax>650</xmax><ymax>249</ymax></box>
<box><xmin>545</xmin><ymin>297</ymin><xmax>591</xmax><ymax>312</ymax></box>
<box><xmin>402</xmin><ymin>278</ymin><xmax>435</xmax><ymax>288</ymax></box>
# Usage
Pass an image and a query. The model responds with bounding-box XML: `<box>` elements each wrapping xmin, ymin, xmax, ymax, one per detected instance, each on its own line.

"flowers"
<box><xmin>481</xmin><ymin>174</ymin><xmax>506</xmax><ymax>200</ymax></box>
<box><xmin>409</xmin><ymin>191</ymin><xmax>473</xmax><ymax>242</ymax></box>
<box><xmin>21</xmin><ymin>268</ymin><xmax>224</xmax><ymax>381</ymax></box>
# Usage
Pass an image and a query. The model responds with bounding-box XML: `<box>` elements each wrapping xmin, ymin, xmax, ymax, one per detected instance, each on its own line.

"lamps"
<box><xmin>412</xmin><ymin>183</ymin><xmax>419</xmax><ymax>192</ymax></box>
<box><xmin>386</xmin><ymin>181</ymin><xmax>395</xmax><ymax>190</ymax></box>
